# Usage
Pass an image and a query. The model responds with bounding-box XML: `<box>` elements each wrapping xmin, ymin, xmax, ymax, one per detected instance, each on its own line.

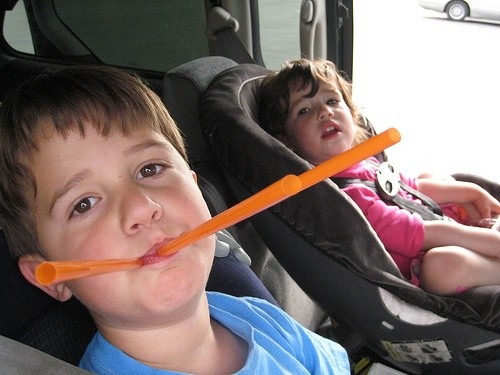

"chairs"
<box><xmin>165</xmin><ymin>59</ymin><xmax>500</xmax><ymax>375</ymax></box>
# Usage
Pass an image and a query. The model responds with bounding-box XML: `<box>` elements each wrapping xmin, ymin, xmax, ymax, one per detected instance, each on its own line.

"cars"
<box><xmin>416</xmin><ymin>0</ymin><xmax>500</xmax><ymax>22</ymax></box>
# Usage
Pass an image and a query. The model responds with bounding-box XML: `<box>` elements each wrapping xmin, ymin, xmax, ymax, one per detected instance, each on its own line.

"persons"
<box><xmin>255</xmin><ymin>59</ymin><xmax>499</xmax><ymax>296</ymax></box>
<box><xmin>0</xmin><ymin>66</ymin><xmax>350</xmax><ymax>375</ymax></box>
